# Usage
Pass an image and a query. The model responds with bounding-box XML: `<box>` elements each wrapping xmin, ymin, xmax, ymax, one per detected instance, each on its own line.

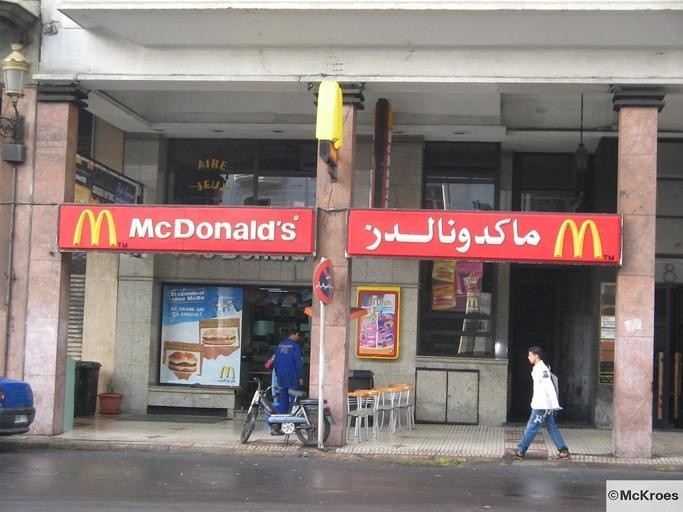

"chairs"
<box><xmin>345</xmin><ymin>383</ymin><xmax>415</xmax><ymax>443</ymax></box>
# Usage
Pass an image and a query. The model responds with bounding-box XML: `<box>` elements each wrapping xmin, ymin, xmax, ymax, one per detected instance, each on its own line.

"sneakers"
<box><xmin>550</xmin><ymin>449</ymin><xmax>570</xmax><ymax>462</ymax></box>
<box><xmin>505</xmin><ymin>448</ymin><xmax>525</xmax><ymax>461</ymax></box>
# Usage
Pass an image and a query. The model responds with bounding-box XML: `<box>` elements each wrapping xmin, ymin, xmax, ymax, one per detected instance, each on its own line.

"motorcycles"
<box><xmin>240</xmin><ymin>378</ymin><xmax>331</xmax><ymax>446</ymax></box>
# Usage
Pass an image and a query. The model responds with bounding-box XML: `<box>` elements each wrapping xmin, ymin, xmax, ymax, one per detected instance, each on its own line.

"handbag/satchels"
<box><xmin>549</xmin><ymin>364</ymin><xmax>559</xmax><ymax>401</ymax></box>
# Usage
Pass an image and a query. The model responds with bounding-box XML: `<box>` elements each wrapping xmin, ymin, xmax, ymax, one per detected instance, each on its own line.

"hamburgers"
<box><xmin>202</xmin><ymin>329</ymin><xmax>237</xmax><ymax>346</ymax></box>
<box><xmin>168</xmin><ymin>352</ymin><xmax>197</xmax><ymax>372</ymax></box>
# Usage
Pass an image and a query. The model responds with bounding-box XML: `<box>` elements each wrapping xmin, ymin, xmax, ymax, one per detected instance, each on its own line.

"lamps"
<box><xmin>2</xmin><ymin>41</ymin><xmax>31</xmax><ymax>142</ymax></box>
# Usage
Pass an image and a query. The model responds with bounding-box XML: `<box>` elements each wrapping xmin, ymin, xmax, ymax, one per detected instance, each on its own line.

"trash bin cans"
<box><xmin>347</xmin><ymin>369</ymin><xmax>374</xmax><ymax>428</ymax></box>
<box><xmin>73</xmin><ymin>361</ymin><xmax>100</xmax><ymax>417</ymax></box>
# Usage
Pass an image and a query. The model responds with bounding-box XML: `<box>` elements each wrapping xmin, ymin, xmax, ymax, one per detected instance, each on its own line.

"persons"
<box><xmin>270</xmin><ymin>329</ymin><xmax>304</xmax><ymax>435</ymax></box>
<box><xmin>264</xmin><ymin>354</ymin><xmax>279</xmax><ymax>403</ymax></box>
<box><xmin>506</xmin><ymin>346</ymin><xmax>570</xmax><ymax>461</ymax></box>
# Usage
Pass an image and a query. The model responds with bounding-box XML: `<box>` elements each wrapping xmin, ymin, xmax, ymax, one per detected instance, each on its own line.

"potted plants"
<box><xmin>96</xmin><ymin>376</ymin><xmax>124</xmax><ymax>414</ymax></box>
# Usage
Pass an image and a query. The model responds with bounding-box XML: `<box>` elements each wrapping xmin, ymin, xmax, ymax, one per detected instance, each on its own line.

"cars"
<box><xmin>0</xmin><ymin>377</ymin><xmax>35</xmax><ymax>435</ymax></box>
